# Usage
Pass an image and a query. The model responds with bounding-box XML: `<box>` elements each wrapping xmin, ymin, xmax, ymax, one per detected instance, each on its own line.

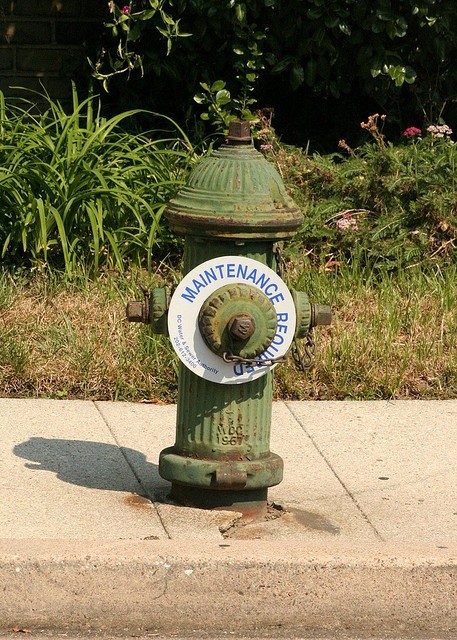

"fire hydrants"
<box><xmin>124</xmin><ymin>121</ymin><xmax>334</xmax><ymax>515</ymax></box>
